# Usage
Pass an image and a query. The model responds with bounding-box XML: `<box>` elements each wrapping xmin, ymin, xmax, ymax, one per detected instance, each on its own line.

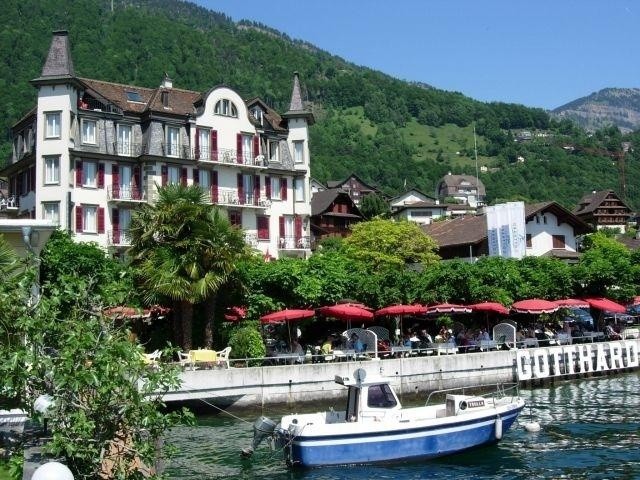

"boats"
<box><xmin>234</xmin><ymin>363</ymin><xmax>534</xmax><ymax>472</ymax></box>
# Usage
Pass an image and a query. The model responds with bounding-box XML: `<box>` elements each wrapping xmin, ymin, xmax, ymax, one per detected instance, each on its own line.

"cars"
<box><xmin>556</xmin><ymin>301</ymin><xmax>640</xmax><ymax>331</ymax></box>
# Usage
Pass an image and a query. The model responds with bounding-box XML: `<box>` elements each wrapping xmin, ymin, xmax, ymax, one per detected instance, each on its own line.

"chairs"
<box><xmin>258</xmin><ymin>195</ymin><xmax>267</xmax><ymax>206</ymax></box>
<box><xmin>298</xmin><ymin>237</ymin><xmax>307</xmax><ymax>249</ymax></box>
<box><xmin>255</xmin><ymin>154</ymin><xmax>265</xmax><ymax>166</ymax></box>
<box><xmin>223</xmin><ymin>150</ymin><xmax>237</xmax><ymax>163</ymax></box>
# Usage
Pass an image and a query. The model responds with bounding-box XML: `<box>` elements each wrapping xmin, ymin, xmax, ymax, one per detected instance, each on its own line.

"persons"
<box><xmin>291</xmin><ymin>338</ymin><xmax>304</xmax><ymax>356</ymax></box>
<box><xmin>323</xmin><ymin>321</ymin><xmax>618</xmax><ymax>362</ymax></box>
